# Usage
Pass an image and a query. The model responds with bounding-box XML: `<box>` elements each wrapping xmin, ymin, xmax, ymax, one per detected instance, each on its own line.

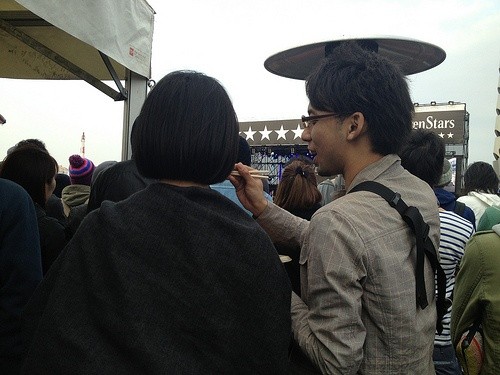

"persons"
<box><xmin>0</xmin><ymin>51</ymin><xmax>500</xmax><ymax>374</ymax></box>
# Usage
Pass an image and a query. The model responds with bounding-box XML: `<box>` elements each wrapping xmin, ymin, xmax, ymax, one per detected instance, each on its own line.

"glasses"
<box><xmin>301</xmin><ymin>113</ymin><xmax>352</xmax><ymax>129</ymax></box>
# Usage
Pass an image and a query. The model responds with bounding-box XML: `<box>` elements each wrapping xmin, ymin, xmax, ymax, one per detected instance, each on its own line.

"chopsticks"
<box><xmin>230</xmin><ymin>169</ymin><xmax>270</xmax><ymax>179</ymax></box>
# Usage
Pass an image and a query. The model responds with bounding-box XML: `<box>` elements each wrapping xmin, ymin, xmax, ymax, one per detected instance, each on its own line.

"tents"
<box><xmin>0</xmin><ymin>1</ymin><xmax>156</xmax><ymax>162</ymax></box>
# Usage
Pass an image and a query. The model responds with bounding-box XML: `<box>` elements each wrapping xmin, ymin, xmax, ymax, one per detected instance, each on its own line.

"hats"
<box><xmin>69</xmin><ymin>154</ymin><xmax>96</xmax><ymax>186</ymax></box>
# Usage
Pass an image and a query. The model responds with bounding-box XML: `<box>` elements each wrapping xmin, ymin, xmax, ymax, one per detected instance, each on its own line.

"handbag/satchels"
<box><xmin>457</xmin><ymin>330</ymin><xmax>483</xmax><ymax>375</ymax></box>
